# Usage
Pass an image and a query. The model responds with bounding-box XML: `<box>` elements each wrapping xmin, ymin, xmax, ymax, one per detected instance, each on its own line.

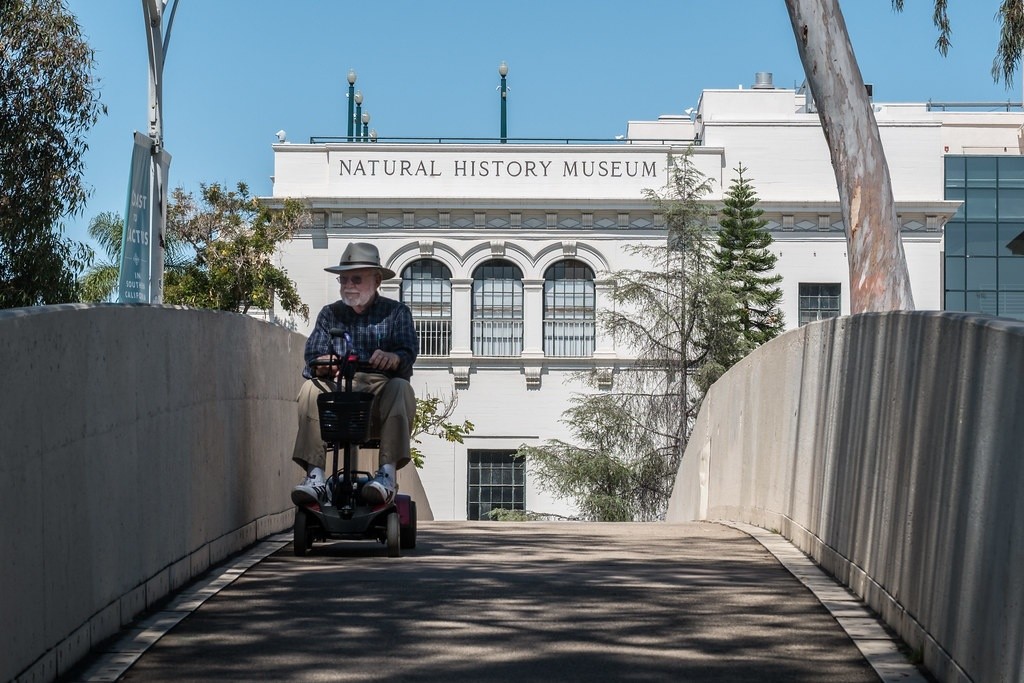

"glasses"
<box><xmin>336</xmin><ymin>276</ymin><xmax>362</xmax><ymax>284</ymax></box>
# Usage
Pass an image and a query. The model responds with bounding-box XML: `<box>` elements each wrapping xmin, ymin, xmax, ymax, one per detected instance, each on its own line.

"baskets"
<box><xmin>316</xmin><ymin>391</ymin><xmax>375</xmax><ymax>442</ymax></box>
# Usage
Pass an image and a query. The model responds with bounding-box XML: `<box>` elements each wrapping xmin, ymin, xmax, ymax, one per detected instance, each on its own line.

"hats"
<box><xmin>323</xmin><ymin>242</ymin><xmax>396</xmax><ymax>280</ymax></box>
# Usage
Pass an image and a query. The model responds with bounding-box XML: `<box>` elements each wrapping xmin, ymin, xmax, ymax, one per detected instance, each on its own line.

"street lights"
<box><xmin>498</xmin><ymin>60</ymin><xmax>509</xmax><ymax>144</ymax></box>
<box><xmin>368</xmin><ymin>128</ymin><xmax>377</xmax><ymax>143</ymax></box>
<box><xmin>355</xmin><ymin>89</ymin><xmax>363</xmax><ymax>143</ymax></box>
<box><xmin>361</xmin><ymin>110</ymin><xmax>370</xmax><ymax>143</ymax></box>
<box><xmin>346</xmin><ymin>67</ymin><xmax>356</xmax><ymax>143</ymax></box>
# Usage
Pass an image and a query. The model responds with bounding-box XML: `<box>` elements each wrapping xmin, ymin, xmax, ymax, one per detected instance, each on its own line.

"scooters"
<box><xmin>293</xmin><ymin>328</ymin><xmax>419</xmax><ymax>556</ymax></box>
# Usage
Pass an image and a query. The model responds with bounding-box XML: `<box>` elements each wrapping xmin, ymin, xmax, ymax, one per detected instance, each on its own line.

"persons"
<box><xmin>290</xmin><ymin>243</ymin><xmax>419</xmax><ymax>509</ymax></box>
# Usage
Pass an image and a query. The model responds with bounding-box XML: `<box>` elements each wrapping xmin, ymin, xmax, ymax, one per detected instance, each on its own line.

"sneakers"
<box><xmin>362</xmin><ymin>466</ymin><xmax>393</xmax><ymax>504</ymax></box>
<box><xmin>291</xmin><ymin>474</ymin><xmax>326</xmax><ymax>507</ymax></box>
